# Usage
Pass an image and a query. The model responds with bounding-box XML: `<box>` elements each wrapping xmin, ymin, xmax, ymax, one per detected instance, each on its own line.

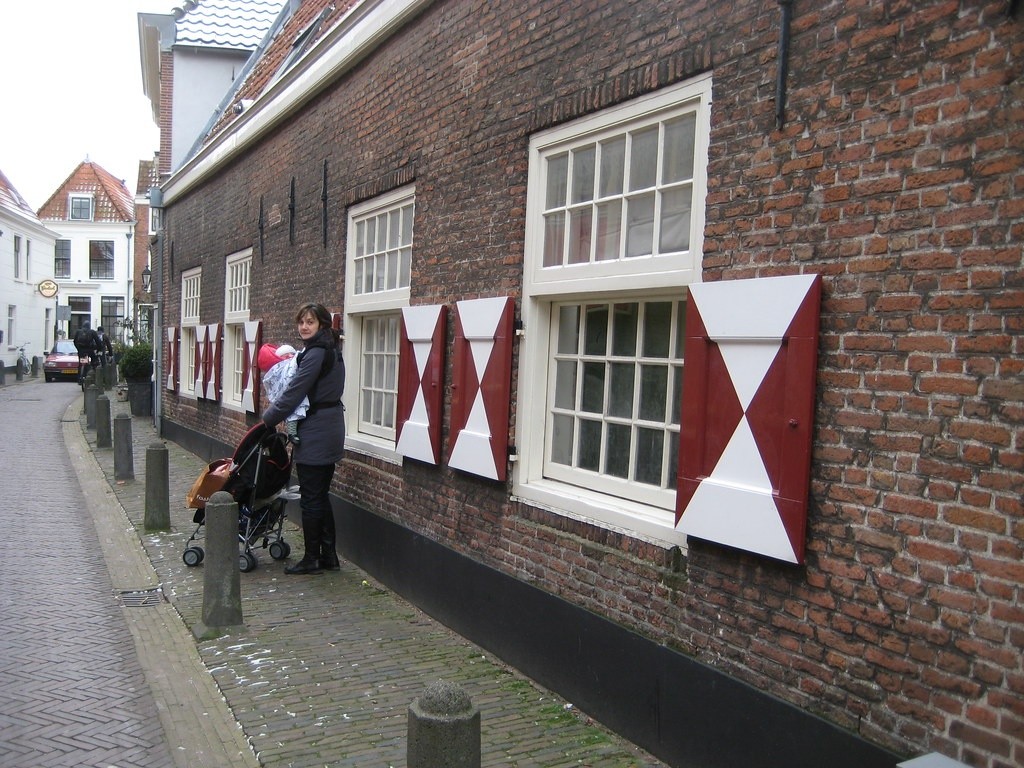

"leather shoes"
<box><xmin>319</xmin><ymin>554</ymin><xmax>339</xmax><ymax>570</ymax></box>
<box><xmin>284</xmin><ymin>558</ymin><xmax>322</xmax><ymax>575</ymax></box>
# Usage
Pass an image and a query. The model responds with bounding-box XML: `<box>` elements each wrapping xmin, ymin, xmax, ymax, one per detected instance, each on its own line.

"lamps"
<box><xmin>141</xmin><ymin>265</ymin><xmax>151</xmax><ymax>293</ymax></box>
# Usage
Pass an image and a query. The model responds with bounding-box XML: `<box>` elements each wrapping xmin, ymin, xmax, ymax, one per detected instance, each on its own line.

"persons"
<box><xmin>74</xmin><ymin>320</ymin><xmax>102</xmax><ymax>385</ymax></box>
<box><xmin>93</xmin><ymin>326</ymin><xmax>113</xmax><ymax>364</ymax></box>
<box><xmin>263</xmin><ymin>300</ymin><xmax>346</xmax><ymax>576</ymax></box>
<box><xmin>257</xmin><ymin>343</ymin><xmax>310</xmax><ymax>445</ymax></box>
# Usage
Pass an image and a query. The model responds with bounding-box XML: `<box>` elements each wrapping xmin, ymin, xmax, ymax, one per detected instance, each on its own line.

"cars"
<box><xmin>42</xmin><ymin>338</ymin><xmax>81</xmax><ymax>382</ymax></box>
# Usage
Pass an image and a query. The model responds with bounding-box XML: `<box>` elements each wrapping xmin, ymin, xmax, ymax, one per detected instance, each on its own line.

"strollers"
<box><xmin>182</xmin><ymin>421</ymin><xmax>302</xmax><ymax>573</ymax></box>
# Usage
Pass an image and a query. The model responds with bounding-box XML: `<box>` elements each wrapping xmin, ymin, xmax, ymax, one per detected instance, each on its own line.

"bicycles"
<box><xmin>16</xmin><ymin>341</ymin><xmax>32</xmax><ymax>375</ymax></box>
<box><xmin>78</xmin><ymin>348</ymin><xmax>114</xmax><ymax>392</ymax></box>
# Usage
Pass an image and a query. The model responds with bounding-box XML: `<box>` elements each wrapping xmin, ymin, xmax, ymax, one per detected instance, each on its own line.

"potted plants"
<box><xmin>117</xmin><ymin>342</ymin><xmax>154</xmax><ymax>417</ymax></box>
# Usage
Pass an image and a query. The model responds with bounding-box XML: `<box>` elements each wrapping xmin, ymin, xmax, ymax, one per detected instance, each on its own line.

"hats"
<box><xmin>275</xmin><ymin>345</ymin><xmax>297</xmax><ymax>358</ymax></box>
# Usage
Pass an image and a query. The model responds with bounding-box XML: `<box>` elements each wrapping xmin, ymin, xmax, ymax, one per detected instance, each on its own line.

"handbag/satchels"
<box><xmin>188</xmin><ymin>459</ymin><xmax>233</xmax><ymax>509</ymax></box>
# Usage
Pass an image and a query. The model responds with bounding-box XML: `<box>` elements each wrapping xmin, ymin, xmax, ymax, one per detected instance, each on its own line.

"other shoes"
<box><xmin>289</xmin><ymin>432</ymin><xmax>301</xmax><ymax>445</ymax></box>
